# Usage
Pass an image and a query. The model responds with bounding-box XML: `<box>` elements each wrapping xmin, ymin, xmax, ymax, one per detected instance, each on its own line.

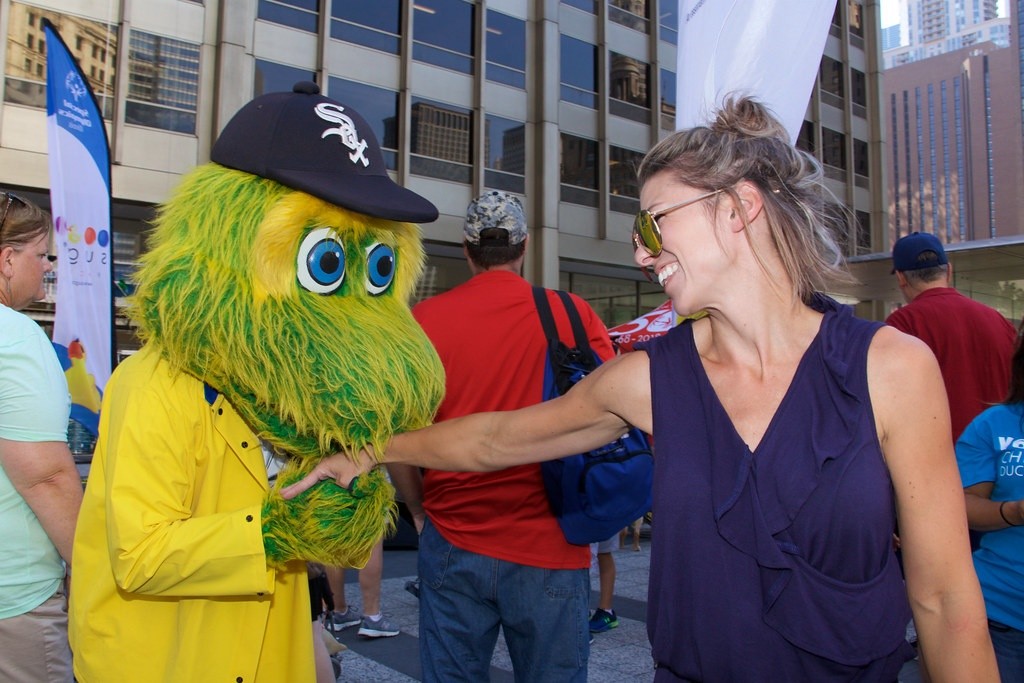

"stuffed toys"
<box><xmin>68</xmin><ymin>79</ymin><xmax>446</xmax><ymax>683</ymax></box>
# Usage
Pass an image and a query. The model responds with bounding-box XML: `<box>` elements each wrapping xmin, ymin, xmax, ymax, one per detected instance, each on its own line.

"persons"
<box><xmin>879</xmin><ymin>232</ymin><xmax>1020</xmax><ymax>662</ymax></box>
<box><xmin>279</xmin><ymin>95</ymin><xmax>1001</xmax><ymax>682</ymax></box>
<box><xmin>589</xmin><ymin>632</ymin><xmax>596</xmax><ymax>644</ymax></box>
<box><xmin>955</xmin><ymin>317</ymin><xmax>1024</xmax><ymax>683</ymax></box>
<box><xmin>328</xmin><ymin>529</ymin><xmax>618</xmax><ymax>645</ymax></box>
<box><xmin>0</xmin><ymin>191</ymin><xmax>84</xmax><ymax>683</ymax></box>
<box><xmin>388</xmin><ymin>187</ymin><xmax>614</xmax><ymax>683</ymax></box>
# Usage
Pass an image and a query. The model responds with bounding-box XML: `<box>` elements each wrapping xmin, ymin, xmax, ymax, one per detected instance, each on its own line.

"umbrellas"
<box><xmin>607</xmin><ymin>299</ymin><xmax>709</xmax><ymax>355</ymax></box>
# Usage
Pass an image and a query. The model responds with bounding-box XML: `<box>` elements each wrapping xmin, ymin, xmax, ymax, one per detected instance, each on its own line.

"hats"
<box><xmin>464</xmin><ymin>190</ymin><xmax>528</xmax><ymax>248</ymax></box>
<box><xmin>891</xmin><ymin>232</ymin><xmax>948</xmax><ymax>275</ymax></box>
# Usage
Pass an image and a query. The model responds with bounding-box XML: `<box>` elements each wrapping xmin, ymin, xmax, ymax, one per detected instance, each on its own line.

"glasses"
<box><xmin>0</xmin><ymin>192</ymin><xmax>28</xmax><ymax>230</ymax></box>
<box><xmin>632</xmin><ymin>185</ymin><xmax>736</xmax><ymax>257</ymax></box>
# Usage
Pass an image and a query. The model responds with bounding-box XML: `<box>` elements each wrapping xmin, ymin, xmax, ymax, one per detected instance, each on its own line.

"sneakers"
<box><xmin>589</xmin><ymin>608</ymin><xmax>618</xmax><ymax>632</ymax></box>
<box><xmin>357</xmin><ymin>613</ymin><xmax>400</xmax><ymax>637</ymax></box>
<box><xmin>327</xmin><ymin>605</ymin><xmax>361</xmax><ymax>631</ymax></box>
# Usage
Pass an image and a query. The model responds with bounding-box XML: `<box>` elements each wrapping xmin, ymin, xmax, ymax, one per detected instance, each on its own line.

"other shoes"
<box><xmin>589</xmin><ymin>634</ymin><xmax>594</xmax><ymax>644</ymax></box>
<box><xmin>632</xmin><ymin>546</ymin><xmax>641</xmax><ymax>551</ymax></box>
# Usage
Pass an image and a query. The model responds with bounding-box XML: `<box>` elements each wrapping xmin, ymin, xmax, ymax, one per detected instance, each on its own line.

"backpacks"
<box><xmin>532</xmin><ymin>284</ymin><xmax>655</xmax><ymax>545</ymax></box>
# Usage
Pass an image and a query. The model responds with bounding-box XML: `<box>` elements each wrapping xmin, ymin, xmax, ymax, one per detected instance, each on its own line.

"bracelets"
<box><xmin>999</xmin><ymin>500</ymin><xmax>1022</xmax><ymax>527</ymax></box>
